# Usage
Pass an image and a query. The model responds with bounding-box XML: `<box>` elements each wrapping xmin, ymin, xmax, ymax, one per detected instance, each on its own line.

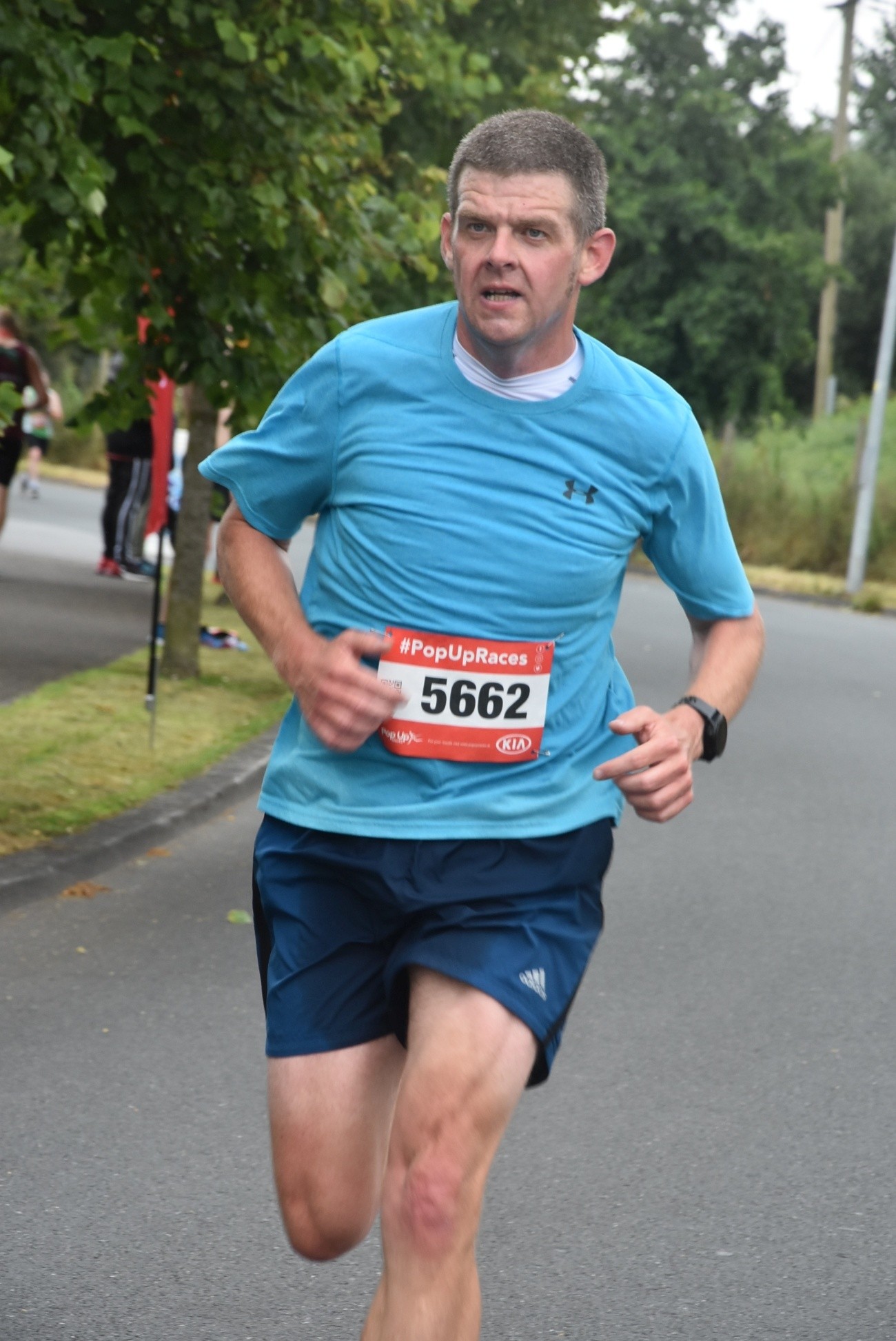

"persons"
<box><xmin>0</xmin><ymin>305</ymin><xmax>64</xmax><ymax>537</ymax></box>
<box><xmin>194</xmin><ymin>108</ymin><xmax>766</xmax><ymax>1340</ymax></box>
<box><xmin>91</xmin><ymin>338</ymin><xmax>253</xmax><ymax>582</ymax></box>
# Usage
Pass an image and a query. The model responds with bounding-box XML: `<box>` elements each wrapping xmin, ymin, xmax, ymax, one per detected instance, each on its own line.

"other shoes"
<box><xmin>119</xmin><ymin>553</ymin><xmax>158</xmax><ymax>583</ymax></box>
<box><xmin>97</xmin><ymin>554</ymin><xmax>120</xmax><ymax>577</ymax></box>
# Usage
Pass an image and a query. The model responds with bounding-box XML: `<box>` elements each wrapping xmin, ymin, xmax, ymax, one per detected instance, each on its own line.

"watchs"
<box><xmin>672</xmin><ymin>696</ymin><xmax>727</xmax><ymax>764</ymax></box>
